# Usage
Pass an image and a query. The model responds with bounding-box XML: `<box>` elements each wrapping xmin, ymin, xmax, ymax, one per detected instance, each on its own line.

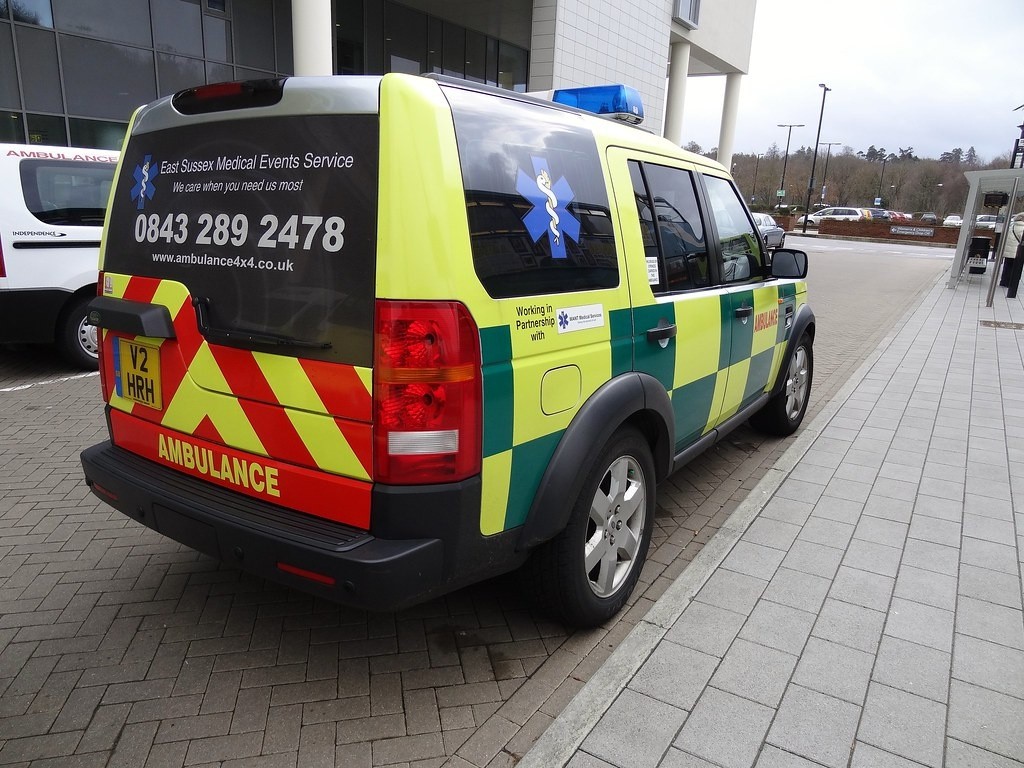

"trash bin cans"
<box><xmin>969</xmin><ymin>235</ymin><xmax>992</xmax><ymax>274</ymax></box>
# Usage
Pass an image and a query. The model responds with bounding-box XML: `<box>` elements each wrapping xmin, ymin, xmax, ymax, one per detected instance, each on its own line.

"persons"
<box><xmin>1000</xmin><ymin>211</ymin><xmax>1024</xmax><ymax>286</ymax></box>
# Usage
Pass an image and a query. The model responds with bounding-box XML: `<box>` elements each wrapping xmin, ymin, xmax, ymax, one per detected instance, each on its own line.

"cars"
<box><xmin>752</xmin><ymin>212</ymin><xmax>785</xmax><ymax>250</ymax></box>
<box><xmin>942</xmin><ymin>216</ymin><xmax>961</xmax><ymax>226</ymax></box>
<box><xmin>857</xmin><ymin>207</ymin><xmax>905</xmax><ymax>223</ymax></box>
<box><xmin>797</xmin><ymin>206</ymin><xmax>865</xmax><ymax>227</ymax></box>
<box><xmin>920</xmin><ymin>214</ymin><xmax>937</xmax><ymax>225</ymax></box>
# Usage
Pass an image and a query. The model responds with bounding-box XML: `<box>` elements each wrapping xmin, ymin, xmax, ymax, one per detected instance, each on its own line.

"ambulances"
<box><xmin>79</xmin><ymin>73</ymin><xmax>811</xmax><ymax>628</ymax></box>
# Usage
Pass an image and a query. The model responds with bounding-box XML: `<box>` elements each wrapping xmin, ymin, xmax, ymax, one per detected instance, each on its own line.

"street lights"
<box><xmin>751</xmin><ymin>153</ymin><xmax>763</xmax><ymax>205</ymax></box>
<box><xmin>878</xmin><ymin>153</ymin><xmax>894</xmax><ymax>197</ymax></box>
<box><xmin>777</xmin><ymin>125</ymin><xmax>805</xmax><ymax>207</ymax></box>
<box><xmin>819</xmin><ymin>143</ymin><xmax>840</xmax><ymax>206</ymax></box>
<box><xmin>802</xmin><ymin>83</ymin><xmax>831</xmax><ymax>233</ymax></box>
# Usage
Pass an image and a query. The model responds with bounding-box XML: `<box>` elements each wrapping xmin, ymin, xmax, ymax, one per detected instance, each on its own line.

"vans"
<box><xmin>959</xmin><ymin>215</ymin><xmax>998</xmax><ymax>229</ymax></box>
<box><xmin>0</xmin><ymin>143</ymin><xmax>124</xmax><ymax>368</ymax></box>
